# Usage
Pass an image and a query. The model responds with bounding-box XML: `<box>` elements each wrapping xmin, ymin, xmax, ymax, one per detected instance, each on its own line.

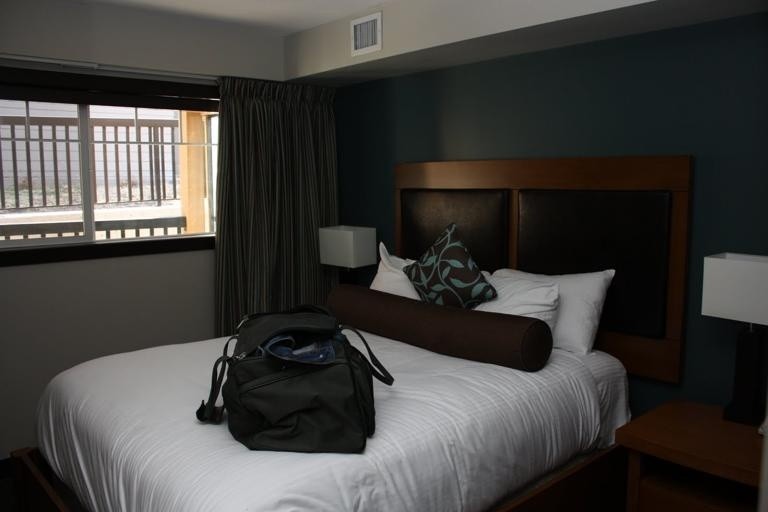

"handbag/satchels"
<box><xmin>195</xmin><ymin>304</ymin><xmax>395</xmax><ymax>454</ymax></box>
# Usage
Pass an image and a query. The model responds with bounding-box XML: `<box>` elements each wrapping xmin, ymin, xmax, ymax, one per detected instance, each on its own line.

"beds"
<box><xmin>35</xmin><ymin>327</ymin><xmax>633</xmax><ymax>512</ymax></box>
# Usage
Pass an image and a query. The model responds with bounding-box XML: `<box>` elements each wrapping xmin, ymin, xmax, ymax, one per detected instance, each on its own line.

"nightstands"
<box><xmin>615</xmin><ymin>399</ymin><xmax>763</xmax><ymax>512</ymax></box>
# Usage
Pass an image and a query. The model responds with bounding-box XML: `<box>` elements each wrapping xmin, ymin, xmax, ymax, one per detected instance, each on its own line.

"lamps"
<box><xmin>317</xmin><ymin>226</ymin><xmax>377</xmax><ymax>283</ymax></box>
<box><xmin>701</xmin><ymin>251</ymin><xmax>768</xmax><ymax>428</ymax></box>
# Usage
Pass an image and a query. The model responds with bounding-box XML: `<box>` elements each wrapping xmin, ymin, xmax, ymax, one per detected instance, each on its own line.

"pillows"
<box><xmin>331</xmin><ymin>282</ymin><xmax>554</xmax><ymax>372</ymax></box>
<box><xmin>402</xmin><ymin>224</ymin><xmax>497</xmax><ymax>310</ymax></box>
<box><xmin>370</xmin><ymin>240</ymin><xmax>422</xmax><ymax>300</ymax></box>
<box><xmin>473</xmin><ymin>268</ymin><xmax>614</xmax><ymax>355</ymax></box>
